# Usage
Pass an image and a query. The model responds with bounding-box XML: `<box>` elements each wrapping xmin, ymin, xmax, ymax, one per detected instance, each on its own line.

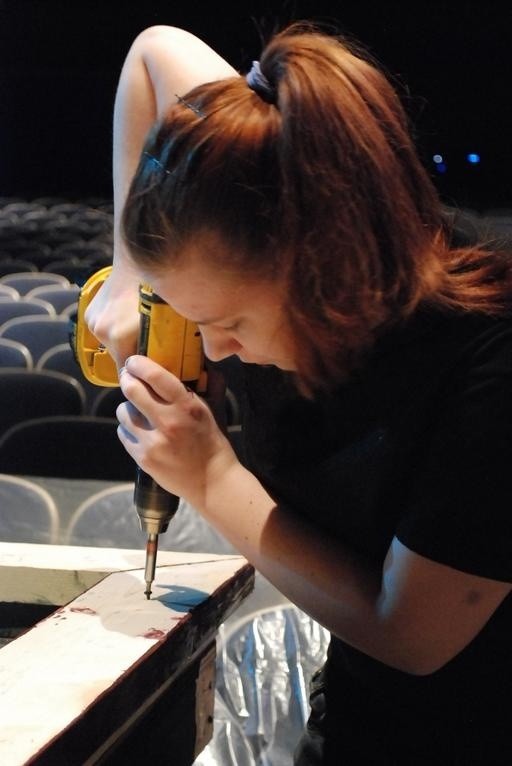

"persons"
<box><xmin>85</xmin><ymin>25</ymin><xmax>511</xmax><ymax>766</ymax></box>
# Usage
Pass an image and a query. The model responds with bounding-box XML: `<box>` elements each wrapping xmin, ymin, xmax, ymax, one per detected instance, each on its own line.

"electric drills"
<box><xmin>67</xmin><ymin>262</ymin><xmax>207</xmax><ymax>601</ymax></box>
